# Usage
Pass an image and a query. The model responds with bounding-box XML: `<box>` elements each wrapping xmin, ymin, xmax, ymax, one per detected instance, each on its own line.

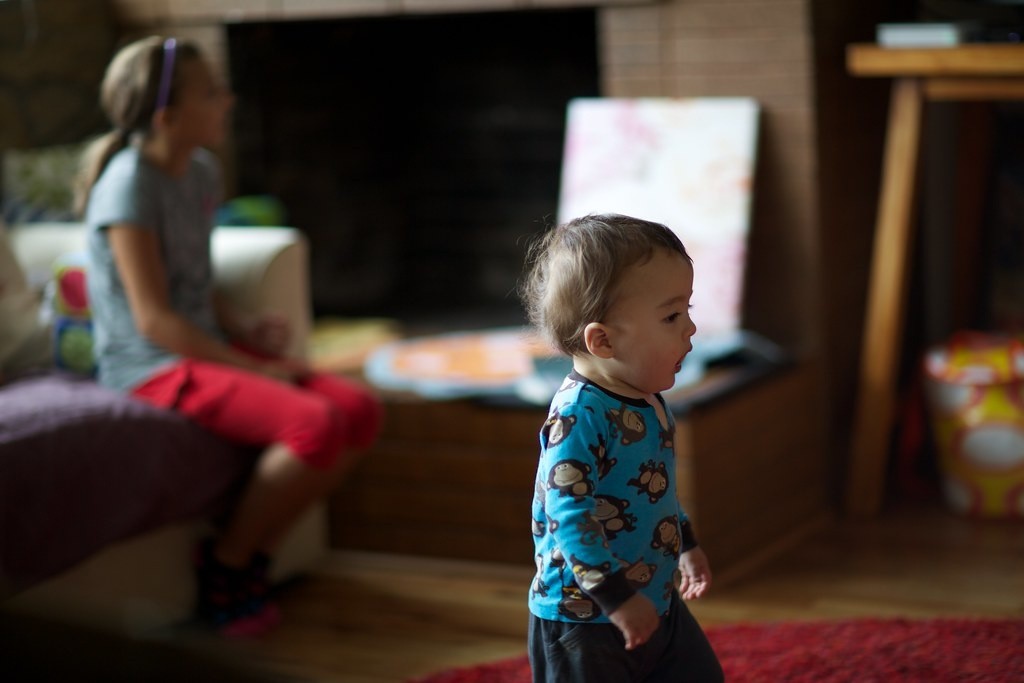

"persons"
<box><xmin>74</xmin><ymin>34</ymin><xmax>384</xmax><ymax>622</ymax></box>
<box><xmin>523</xmin><ymin>213</ymin><xmax>727</xmax><ymax>683</ymax></box>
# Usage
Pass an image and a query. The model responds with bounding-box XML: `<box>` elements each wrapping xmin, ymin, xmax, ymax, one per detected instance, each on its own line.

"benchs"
<box><xmin>324</xmin><ymin>326</ymin><xmax>824</xmax><ymax>594</ymax></box>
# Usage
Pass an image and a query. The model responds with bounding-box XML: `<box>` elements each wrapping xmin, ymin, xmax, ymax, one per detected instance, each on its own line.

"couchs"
<box><xmin>1</xmin><ymin>198</ymin><xmax>309</xmax><ymax>589</ymax></box>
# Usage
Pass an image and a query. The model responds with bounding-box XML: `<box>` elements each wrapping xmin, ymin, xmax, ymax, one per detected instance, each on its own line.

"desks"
<box><xmin>836</xmin><ymin>45</ymin><xmax>1024</xmax><ymax>528</ymax></box>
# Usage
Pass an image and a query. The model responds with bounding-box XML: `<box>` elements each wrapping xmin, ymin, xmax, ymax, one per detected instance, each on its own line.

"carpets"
<box><xmin>412</xmin><ymin>615</ymin><xmax>1024</xmax><ymax>683</ymax></box>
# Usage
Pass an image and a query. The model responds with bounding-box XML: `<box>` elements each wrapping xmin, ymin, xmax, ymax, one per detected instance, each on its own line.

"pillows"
<box><xmin>42</xmin><ymin>260</ymin><xmax>100</xmax><ymax>375</ymax></box>
<box><xmin>2</xmin><ymin>226</ymin><xmax>56</xmax><ymax>381</ymax></box>
